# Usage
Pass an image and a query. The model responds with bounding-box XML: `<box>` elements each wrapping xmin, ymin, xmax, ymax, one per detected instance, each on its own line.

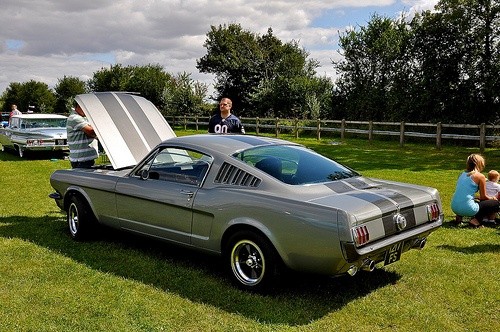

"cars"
<box><xmin>0</xmin><ymin>114</ymin><xmax>71</xmax><ymax>159</ymax></box>
<box><xmin>48</xmin><ymin>91</ymin><xmax>447</xmax><ymax>293</ymax></box>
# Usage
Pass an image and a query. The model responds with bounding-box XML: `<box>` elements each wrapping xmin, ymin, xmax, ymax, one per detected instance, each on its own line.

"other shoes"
<box><xmin>468</xmin><ymin>222</ymin><xmax>482</xmax><ymax>228</ymax></box>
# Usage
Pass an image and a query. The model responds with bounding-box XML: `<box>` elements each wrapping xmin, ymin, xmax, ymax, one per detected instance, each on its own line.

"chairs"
<box><xmin>254</xmin><ymin>158</ymin><xmax>283</xmax><ymax>179</ymax></box>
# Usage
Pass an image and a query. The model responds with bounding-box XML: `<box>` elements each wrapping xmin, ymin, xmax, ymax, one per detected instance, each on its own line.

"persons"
<box><xmin>66</xmin><ymin>99</ymin><xmax>99</xmax><ymax>168</ymax></box>
<box><xmin>451</xmin><ymin>154</ymin><xmax>500</xmax><ymax>226</ymax></box>
<box><xmin>486</xmin><ymin>170</ymin><xmax>500</xmax><ymax>221</ymax></box>
<box><xmin>9</xmin><ymin>105</ymin><xmax>23</xmax><ymax>127</ymax></box>
<box><xmin>208</xmin><ymin>98</ymin><xmax>246</xmax><ymax>135</ymax></box>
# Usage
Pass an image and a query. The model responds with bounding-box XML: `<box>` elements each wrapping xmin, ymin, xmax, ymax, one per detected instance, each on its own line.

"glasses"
<box><xmin>220</xmin><ymin>103</ymin><xmax>227</xmax><ymax>106</ymax></box>
